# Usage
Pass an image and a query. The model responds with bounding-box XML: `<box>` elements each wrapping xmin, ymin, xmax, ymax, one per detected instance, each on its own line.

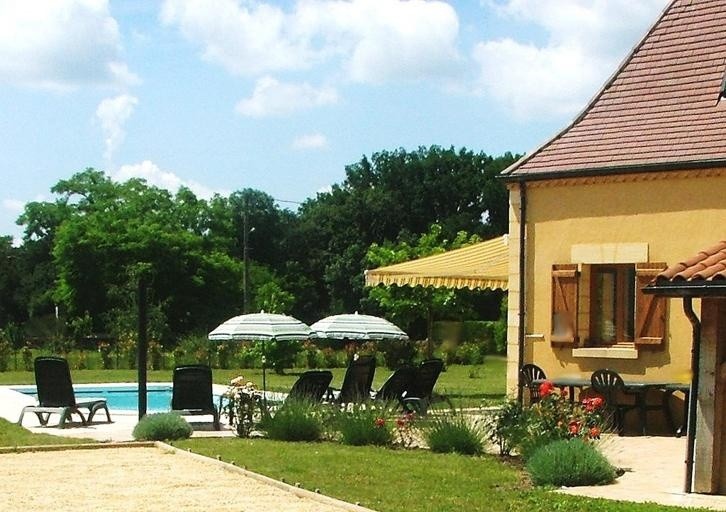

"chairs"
<box><xmin>169</xmin><ymin>363</ymin><xmax>221</xmax><ymax>431</ymax></box>
<box><xmin>253</xmin><ymin>349</ymin><xmax>457</xmax><ymax>427</ymax></box>
<box><xmin>16</xmin><ymin>354</ymin><xmax>114</xmax><ymax>428</ymax></box>
<box><xmin>519</xmin><ymin>363</ymin><xmax>647</xmax><ymax>437</ymax></box>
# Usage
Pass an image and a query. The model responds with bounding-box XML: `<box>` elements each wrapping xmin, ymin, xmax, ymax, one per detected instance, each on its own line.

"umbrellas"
<box><xmin>207</xmin><ymin>311</ymin><xmax>319</xmax><ymax>410</ymax></box>
<box><xmin>310</xmin><ymin>311</ymin><xmax>409</xmax><ymax>355</ymax></box>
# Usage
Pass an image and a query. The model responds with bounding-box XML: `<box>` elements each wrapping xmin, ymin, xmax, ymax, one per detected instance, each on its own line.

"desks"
<box><xmin>533</xmin><ymin>374</ymin><xmax>691</xmax><ymax>438</ymax></box>
